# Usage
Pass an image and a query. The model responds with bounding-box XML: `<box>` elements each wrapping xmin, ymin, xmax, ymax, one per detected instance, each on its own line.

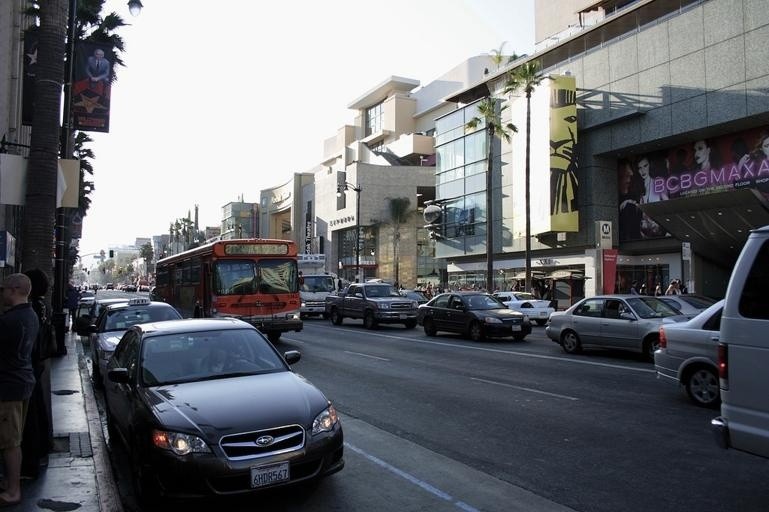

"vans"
<box><xmin>705</xmin><ymin>222</ymin><xmax>769</xmax><ymax>462</ymax></box>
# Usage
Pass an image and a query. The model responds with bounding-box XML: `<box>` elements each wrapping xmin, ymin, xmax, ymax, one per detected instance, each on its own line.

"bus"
<box><xmin>152</xmin><ymin>237</ymin><xmax>304</xmax><ymax>347</ymax></box>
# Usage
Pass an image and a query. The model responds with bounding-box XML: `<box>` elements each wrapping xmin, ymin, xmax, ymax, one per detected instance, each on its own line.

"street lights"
<box><xmin>334</xmin><ymin>179</ymin><xmax>362</xmax><ymax>284</ymax></box>
<box><xmin>53</xmin><ymin>0</ymin><xmax>145</xmax><ymax>361</ymax></box>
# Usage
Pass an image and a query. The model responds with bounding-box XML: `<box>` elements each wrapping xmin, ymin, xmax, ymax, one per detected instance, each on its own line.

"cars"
<box><xmin>542</xmin><ymin>289</ymin><xmax>725</xmax><ymax>413</ymax></box>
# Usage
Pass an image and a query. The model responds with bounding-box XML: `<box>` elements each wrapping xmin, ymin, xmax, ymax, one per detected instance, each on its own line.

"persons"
<box><xmin>86</xmin><ymin>49</ymin><xmax>110</xmax><ymax>89</ymax></box>
<box><xmin>19</xmin><ymin>267</ymin><xmax>50</xmax><ymax>481</ymax></box>
<box><xmin>399</xmin><ymin>279</ymin><xmax>688</xmax><ymax>296</ymax></box>
<box><xmin>619</xmin><ymin>136</ymin><xmax>769</xmax><ymax>240</ymax></box>
<box><xmin>0</xmin><ymin>273</ymin><xmax>40</xmax><ymax>507</ymax></box>
